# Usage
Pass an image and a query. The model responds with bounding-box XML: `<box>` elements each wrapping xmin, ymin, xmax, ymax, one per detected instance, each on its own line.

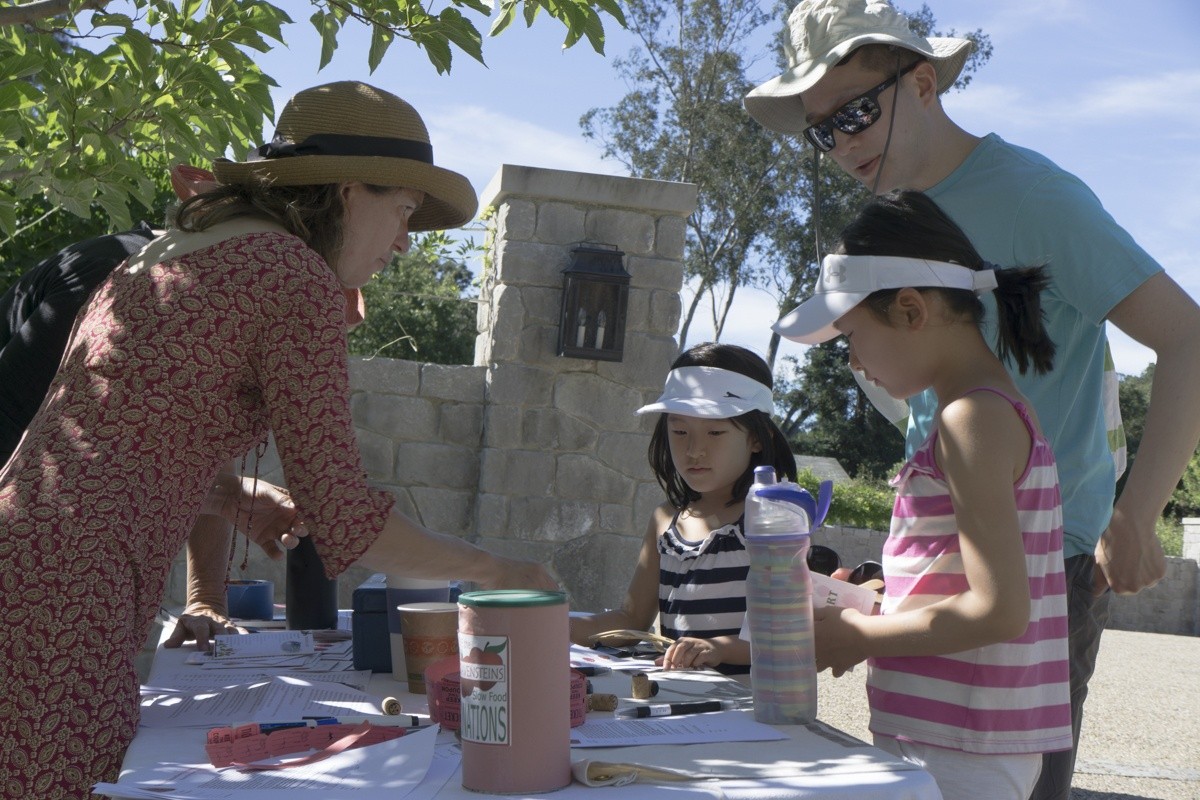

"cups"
<box><xmin>457</xmin><ymin>589</ymin><xmax>573</xmax><ymax>795</ymax></box>
<box><xmin>396</xmin><ymin>601</ymin><xmax>458</xmax><ymax>694</ymax></box>
<box><xmin>286</xmin><ymin>533</ymin><xmax>338</xmax><ymax>630</ymax></box>
<box><xmin>385</xmin><ymin>574</ymin><xmax>451</xmax><ymax>681</ymax></box>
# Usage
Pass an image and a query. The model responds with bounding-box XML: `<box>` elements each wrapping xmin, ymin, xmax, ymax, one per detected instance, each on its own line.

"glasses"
<box><xmin>803</xmin><ymin>60</ymin><xmax>924</xmax><ymax>153</ymax></box>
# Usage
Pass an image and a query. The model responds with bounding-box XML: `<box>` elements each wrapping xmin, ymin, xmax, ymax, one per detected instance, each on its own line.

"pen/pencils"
<box><xmin>618</xmin><ymin>701</ymin><xmax>725</xmax><ymax>718</ymax></box>
<box><xmin>302</xmin><ymin>715</ymin><xmax>406</xmax><ymax>721</ymax></box>
<box><xmin>563</xmin><ymin>667</ymin><xmax>611</xmax><ymax>675</ymax></box>
<box><xmin>405</xmin><ymin>725</ymin><xmax>441</xmax><ymax>733</ymax></box>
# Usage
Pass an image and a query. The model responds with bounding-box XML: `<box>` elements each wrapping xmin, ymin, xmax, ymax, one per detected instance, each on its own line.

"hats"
<box><xmin>743</xmin><ymin>1</ymin><xmax>974</xmax><ymax>134</ymax></box>
<box><xmin>210</xmin><ymin>80</ymin><xmax>478</xmax><ymax>233</ymax></box>
<box><xmin>637</xmin><ymin>366</ymin><xmax>775</xmax><ymax>419</ymax></box>
<box><xmin>171</xmin><ymin>163</ymin><xmax>239</xmax><ymax>219</ymax></box>
<box><xmin>772</xmin><ymin>254</ymin><xmax>998</xmax><ymax>345</ymax></box>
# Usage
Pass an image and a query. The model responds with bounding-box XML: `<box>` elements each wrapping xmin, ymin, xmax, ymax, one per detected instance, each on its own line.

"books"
<box><xmin>738</xmin><ymin>562</ymin><xmax>878</xmax><ymax>673</ymax></box>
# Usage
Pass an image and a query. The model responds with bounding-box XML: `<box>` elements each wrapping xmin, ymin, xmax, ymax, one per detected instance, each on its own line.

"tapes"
<box><xmin>224</xmin><ymin>579</ymin><xmax>273</xmax><ymax>619</ymax></box>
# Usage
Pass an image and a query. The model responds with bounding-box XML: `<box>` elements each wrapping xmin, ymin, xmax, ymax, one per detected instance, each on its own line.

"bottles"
<box><xmin>743</xmin><ymin>465</ymin><xmax>833</xmax><ymax>726</ymax></box>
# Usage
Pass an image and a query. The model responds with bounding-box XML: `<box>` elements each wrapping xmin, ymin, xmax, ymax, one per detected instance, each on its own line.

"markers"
<box><xmin>318</xmin><ymin>712</ymin><xmax>419</xmax><ymax>726</ymax></box>
<box><xmin>232</xmin><ymin>719</ymin><xmax>318</xmax><ymax>730</ymax></box>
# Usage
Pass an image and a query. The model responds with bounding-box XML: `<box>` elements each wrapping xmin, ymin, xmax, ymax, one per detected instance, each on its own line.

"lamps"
<box><xmin>556</xmin><ymin>241</ymin><xmax>633</xmax><ymax>361</ymax></box>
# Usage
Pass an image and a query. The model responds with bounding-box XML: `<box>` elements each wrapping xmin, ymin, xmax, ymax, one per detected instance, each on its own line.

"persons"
<box><xmin>0</xmin><ymin>161</ymin><xmax>250</xmax><ymax>648</ymax></box>
<box><xmin>563</xmin><ymin>344</ymin><xmax>801</xmax><ymax>691</ymax></box>
<box><xmin>0</xmin><ymin>82</ymin><xmax>559</xmax><ymax>800</ymax></box>
<box><xmin>767</xmin><ymin>191</ymin><xmax>1072</xmax><ymax>800</ymax></box>
<box><xmin>746</xmin><ymin>0</ymin><xmax>1200</xmax><ymax>800</ymax></box>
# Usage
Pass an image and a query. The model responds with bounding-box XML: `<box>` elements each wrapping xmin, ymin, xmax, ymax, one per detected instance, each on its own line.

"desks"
<box><xmin>115</xmin><ymin>603</ymin><xmax>942</xmax><ymax>800</ymax></box>
